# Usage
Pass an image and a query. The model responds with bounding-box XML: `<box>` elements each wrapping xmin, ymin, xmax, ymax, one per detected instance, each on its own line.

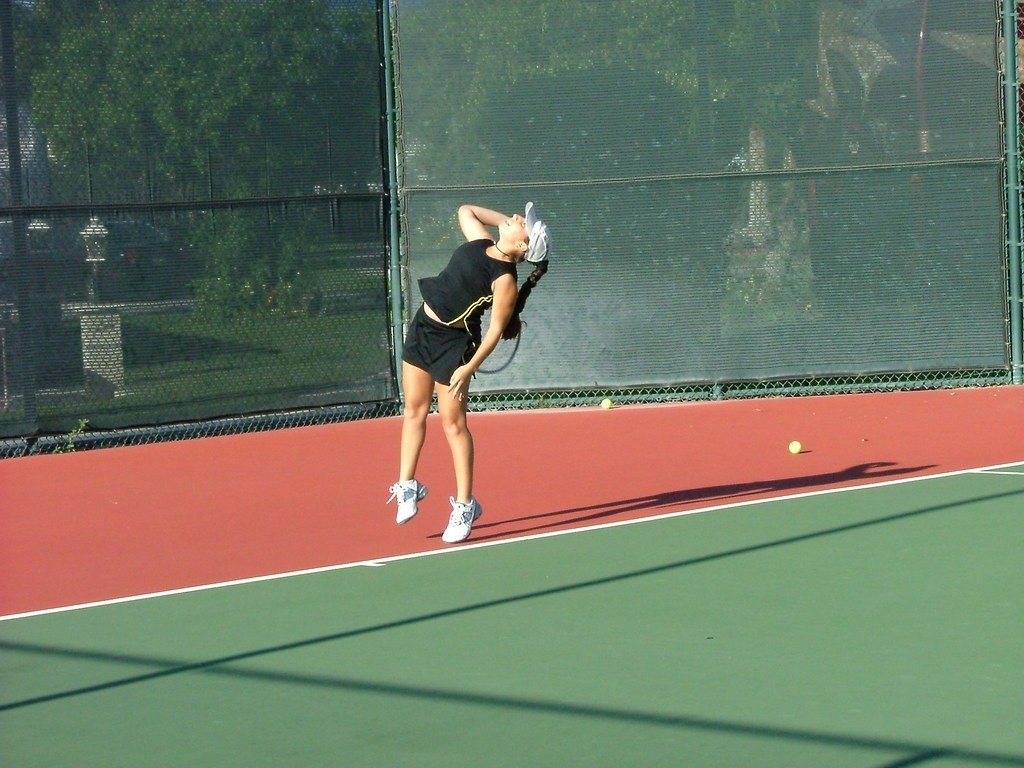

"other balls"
<box><xmin>601</xmin><ymin>399</ymin><xmax>612</xmax><ymax>409</ymax></box>
<box><xmin>788</xmin><ymin>440</ymin><xmax>801</xmax><ymax>454</ymax></box>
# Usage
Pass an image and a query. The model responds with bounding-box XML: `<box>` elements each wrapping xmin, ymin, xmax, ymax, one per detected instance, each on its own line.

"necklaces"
<box><xmin>494</xmin><ymin>241</ymin><xmax>510</xmax><ymax>257</ymax></box>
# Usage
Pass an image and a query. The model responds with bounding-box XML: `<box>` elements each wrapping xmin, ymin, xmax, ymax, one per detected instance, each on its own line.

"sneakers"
<box><xmin>442</xmin><ymin>496</ymin><xmax>482</xmax><ymax>544</ymax></box>
<box><xmin>385</xmin><ymin>479</ymin><xmax>428</xmax><ymax>525</ymax></box>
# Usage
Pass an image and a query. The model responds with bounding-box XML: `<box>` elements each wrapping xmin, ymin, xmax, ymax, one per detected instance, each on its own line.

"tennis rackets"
<box><xmin>472</xmin><ymin>306</ymin><xmax>522</xmax><ymax>376</ymax></box>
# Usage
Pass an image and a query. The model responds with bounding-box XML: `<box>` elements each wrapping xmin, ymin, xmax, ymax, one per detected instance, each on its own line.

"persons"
<box><xmin>387</xmin><ymin>201</ymin><xmax>552</xmax><ymax>542</ymax></box>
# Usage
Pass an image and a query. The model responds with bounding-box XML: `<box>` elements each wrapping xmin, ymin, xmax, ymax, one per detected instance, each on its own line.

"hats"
<box><xmin>524</xmin><ymin>201</ymin><xmax>550</xmax><ymax>263</ymax></box>
